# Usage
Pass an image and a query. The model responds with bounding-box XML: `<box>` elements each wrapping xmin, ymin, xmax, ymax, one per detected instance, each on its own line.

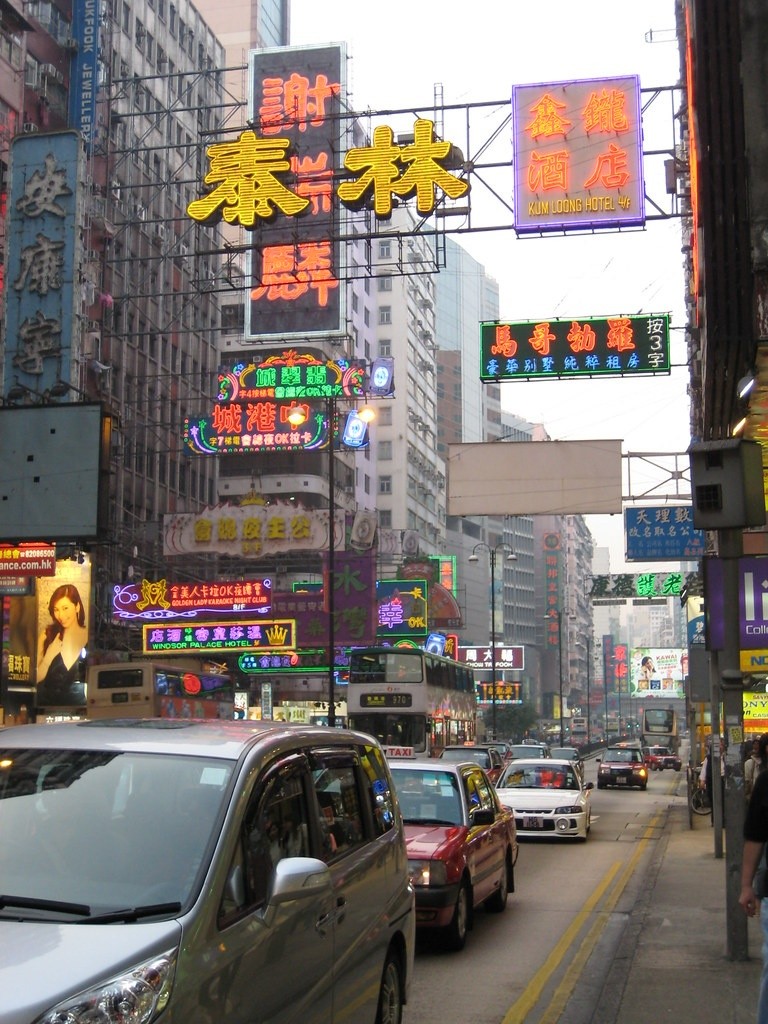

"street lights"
<box><xmin>287</xmin><ymin>382</ymin><xmax>376</xmax><ymax>728</ymax></box>
<box><xmin>469</xmin><ymin>542</ymin><xmax>518</xmax><ymax>740</ymax></box>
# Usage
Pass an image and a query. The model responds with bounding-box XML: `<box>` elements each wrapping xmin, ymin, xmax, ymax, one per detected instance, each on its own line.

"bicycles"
<box><xmin>689</xmin><ymin>774</ymin><xmax>712</xmax><ymax>816</ymax></box>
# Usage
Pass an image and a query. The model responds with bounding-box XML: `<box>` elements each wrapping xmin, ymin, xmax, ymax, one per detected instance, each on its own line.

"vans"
<box><xmin>1</xmin><ymin>715</ymin><xmax>418</xmax><ymax>1024</ymax></box>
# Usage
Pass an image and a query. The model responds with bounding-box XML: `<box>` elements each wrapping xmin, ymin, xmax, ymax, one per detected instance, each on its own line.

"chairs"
<box><xmin>109</xmin><ymin>771</ymin><xmax>197</xmax><ymax>878</ymax></box>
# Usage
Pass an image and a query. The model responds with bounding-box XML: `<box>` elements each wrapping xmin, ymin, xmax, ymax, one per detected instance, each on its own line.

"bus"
<box><xmin>86</xmin><ymin>662</ymin><xmax>237</xmax><ymax>721</ymax></box>
<box><xmin>345</xmin><ymin>644</ymin><xmax>480</xmax><ymax>758</ymax></box>
<box><xmin>640</xmin><ymin>707</ymin><xmax>679</xmax><ymax>755</ymax></box>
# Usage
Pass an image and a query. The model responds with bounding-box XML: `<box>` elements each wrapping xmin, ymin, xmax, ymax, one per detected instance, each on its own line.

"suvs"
<box><xmin>439</xmin><ymin>744</ymin><xmax>505</xmax><ymax>787</ymax></box>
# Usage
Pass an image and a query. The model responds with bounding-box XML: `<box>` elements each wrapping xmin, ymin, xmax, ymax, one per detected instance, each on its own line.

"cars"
<box><xmin>493</xmin><ymin>756</ymin><xmax>594</xmax><ymax>843</ymax></box>
<box><xmin>595</xmin><ymin>743</ymin><xmax>649</xmax><ymax>791</ymax></box>
<box><xmin>642</xmin><ymin>745</ymin><xmax>682</xmax><ymax>772</ymax></box>
<box><xmin>316</xmin><ymin>742</ymin><xmax>520</xmax><ymax>953</ymax></box>
<box><xmin>482</xmin><ymin>715</ymin><xmax>626</xmax><ymax>782</ymax></box>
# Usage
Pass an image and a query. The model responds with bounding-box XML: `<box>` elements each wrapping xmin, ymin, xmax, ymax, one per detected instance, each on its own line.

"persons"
<box><xmin>546</xmin><ymin>733</ymin><xmax>559</xmax><ymax>742</ymax></box>
<box><xmin>37</xmin><ymin>584</ymin><xmax>88</xmax><ymax>705</ymax></box>
<box><xmin>699</xmin><ymin>732</ymin><xmax>768</xmax><ymax>1024</ymax></box>
<box><xmin>642</xmin><ymin>656</ymin><xmax>655</xmax><ymax>678</ymax></box>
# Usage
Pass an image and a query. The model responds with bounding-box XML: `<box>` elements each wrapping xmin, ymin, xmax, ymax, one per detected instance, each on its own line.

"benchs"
<box><xmin>401</xmin><ymin>794</ymin><xmax>455</xmax><ymax>820</ymax></box>
